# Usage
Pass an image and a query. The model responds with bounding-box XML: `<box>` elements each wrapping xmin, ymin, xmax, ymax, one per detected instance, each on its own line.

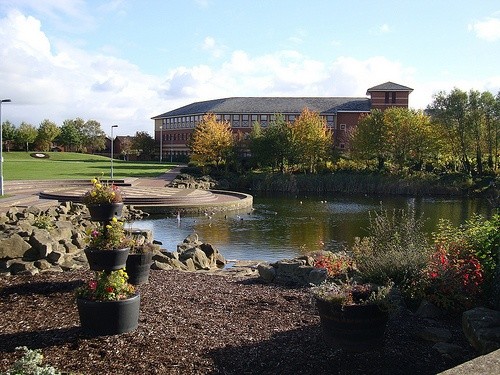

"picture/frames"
<box><xmin>123</xmin><ymin>230</ymin><xmax>160</xmax><ymax>254</ymax></box>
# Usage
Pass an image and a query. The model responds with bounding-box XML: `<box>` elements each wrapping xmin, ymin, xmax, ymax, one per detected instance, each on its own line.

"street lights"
<box><xmin>0</xmin><ymin>98</ymin><xmax>12</xmax><ymax>195</ymax></box>
<box><xmin>159</xmin><ymin>125</ymin><xmax>163</xmax><ymax>162</ymax></box>
<box><xmin>110</xmin><ymin>124</ymin><xmax>118</xmax><ymax>179</ymax></box>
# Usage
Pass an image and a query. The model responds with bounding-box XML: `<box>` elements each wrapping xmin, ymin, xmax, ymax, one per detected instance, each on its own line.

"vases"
<box><xmin>125</xmin><ymin>252</ymin><xmax>152</xmax><ymax>284</ymax></box>
<box><xmin>316</xmin><ymin>300</ymin><xmax>387</xmax><ymax>353</ymax></box>
<box><xmin>74</xmin><ymin>293</ymin><xmax>141</xmax><ymax>337</ymax></box>
<box><xmin>84</xmin><ymin>248</ymin><xmax>130</xmax><ymax>272</ymax></box>
<box><xmin>86</xmin><ymin>203</ymin><xmax>124</xmax><ymax>220</ymax></box>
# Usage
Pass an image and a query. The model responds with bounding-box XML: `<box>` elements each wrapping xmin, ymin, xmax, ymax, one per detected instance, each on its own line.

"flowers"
<box><xmin>82</xmin><ymin>215</ymin><xmax>136</xmax><ymax>252</ymax></box>
<box><xmin>71</xmin><ymin>269</ymin><xmax>138</xmax><ymax>304</ymax></box>
<box><xmin>307</xmin><ymin>273</ymin><xmax>408</xmax><ymax>315</ymax></box>
<box><xmin>84</xmin><ymin>177</ymin><xmax>122</xmax><ymax>204</ymax></box>
